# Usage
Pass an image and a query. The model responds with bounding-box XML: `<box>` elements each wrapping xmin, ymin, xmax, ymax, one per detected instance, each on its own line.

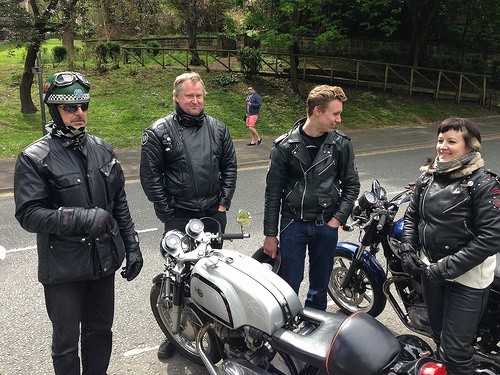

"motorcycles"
<box><xmin>327</xmin><ymin>180</ymin><xmax>499</xmax><ymax>373</ymax></box>
<box><xmin>149</xmin><ymin>209</ymin><xmax>447</xmax><ymax>375</ymax></box>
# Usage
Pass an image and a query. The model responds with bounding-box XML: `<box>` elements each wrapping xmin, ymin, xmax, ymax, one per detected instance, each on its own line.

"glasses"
<box><xmin>57</xmin><ymin>103</ymin><xmax>88</xmax><ymax>113</ymax></box>
<box><xmin>53</xmin><ymin>72</ymin><xmax>91</xmax><ymax>91</ymax></box>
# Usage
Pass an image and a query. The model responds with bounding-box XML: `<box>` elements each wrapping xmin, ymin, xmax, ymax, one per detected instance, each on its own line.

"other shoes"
<box><xmin>157</xmin><ymin>338</ymin><xmax>177</xmax><ymax>358</ymax></box>
<box><xmin>247</xmin><ymin>142</ymin><xmax>256</xmax><ymax>146</ymax></box>
<box><xmin>258</xmin><ymin>138</ymin><xmax>262</xmax><ymax>145</ymax></box>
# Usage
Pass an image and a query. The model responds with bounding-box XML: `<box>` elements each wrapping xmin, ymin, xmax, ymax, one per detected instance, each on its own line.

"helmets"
<box><xmin>44</xmin><ymin>82</ymin><xmax>90</xmax><ymax>104</ymax></box>
<box><xmin>252</xmin><ymin>245</ymin><xmax>282</xmax><ymax>275</ymax></box>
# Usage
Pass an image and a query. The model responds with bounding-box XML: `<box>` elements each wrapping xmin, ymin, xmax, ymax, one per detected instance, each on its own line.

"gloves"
<box><xmin>400</xmin><ymin>251</ymin><xmax>428</xmax><ymax>284</ymax></box>
<box><xmin>57</xmin><ymin>206</ymin><xmax>114</xmax><ymax>240</ymax></box>
<box><xmin>421</xmin><ymin>263</ymin><xmax>445</xmax><ymax>292</ymax></box>
<box><xmin>121</xmin><ymin>233</ymin><xmax>143</xmax><ymax>281</ymax></box>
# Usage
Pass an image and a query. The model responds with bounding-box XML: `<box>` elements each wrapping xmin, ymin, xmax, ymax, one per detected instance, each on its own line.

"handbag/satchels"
<box><xmin>244</xmin><ymin>115</ymin><xmax>246</xmax><ymax>122</ymax></box>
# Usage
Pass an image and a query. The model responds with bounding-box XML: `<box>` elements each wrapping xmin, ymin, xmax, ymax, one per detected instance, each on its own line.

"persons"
<box><xmin>244</xmin><ymin>85</ymin><xmax>262</xmax><ymax>146</ymax></box>
<box><xmin>399</xmin><ymin>118</ymin><xmax>500</xmax><ymax>375</ymax></box>
<box><xmin>13</xmin><ymin>71</ymin><xmax>143</xmax><ymax>375</ymax></box>
<box><xmin>262</xmin><ymin>85</ymin><xmax>360</xmax><ymax>311</ymax></box>
<box><xmin>139</xmin><ymin>72</ymin><xmax>238</xmax><ymax>357</ymax></box>
